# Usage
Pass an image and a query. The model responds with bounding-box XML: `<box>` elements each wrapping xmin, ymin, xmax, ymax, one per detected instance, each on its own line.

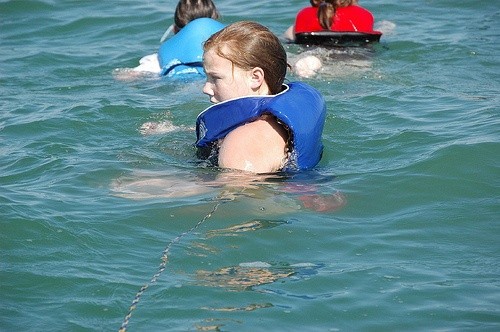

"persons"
<box><xmin>285</xmin><ymin>0</ymin><xmax>383</xmax><ymax>79</ymax></box>
<box><xmin>111</xmin><ymin>20</ymin><xmax>328</xmax><ymax>197</ymax></box>
<box><xmin>117</xmin><ymin>0</ymin><xmax>231</xmax><ymax>83</ymax></box>
